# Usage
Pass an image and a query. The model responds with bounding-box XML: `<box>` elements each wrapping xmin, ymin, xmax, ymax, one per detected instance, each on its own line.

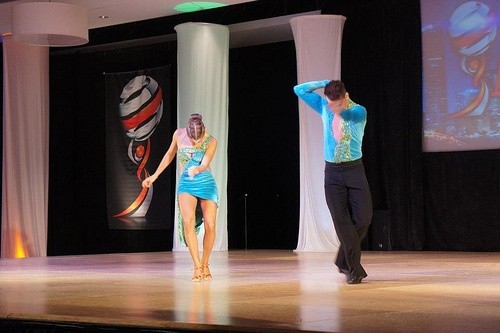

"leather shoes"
<box><xmin>337</xmin><ymin>265</ymin><xmax>348</xmax><ymax>274</ymax></box>
<box><xmin>347</xmin><ymin>266</ymin><xmax>368</xmax><ymax>283</ymax></box>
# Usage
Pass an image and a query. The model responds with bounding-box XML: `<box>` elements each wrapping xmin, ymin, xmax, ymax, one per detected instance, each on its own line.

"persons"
<box><xmin>293</xmin><ymin>78</ymin><xmax>374</xmax><ymax>284</ymax></box>
<box><xmin>142</xmin><ymin>113</ymin><xmax>219</xmax><ymax>283</ymax></box>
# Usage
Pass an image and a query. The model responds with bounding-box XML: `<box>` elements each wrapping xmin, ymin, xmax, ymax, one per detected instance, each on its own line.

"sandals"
<box><xmin>201</xmin><ymin>264</ymin><xmax>212</xmax><ymax>280</ymax></box>
<box><xmin>191</xmin><ymin>264</ymin><xmax>202</xmax><ymax>282</ymax></box>
